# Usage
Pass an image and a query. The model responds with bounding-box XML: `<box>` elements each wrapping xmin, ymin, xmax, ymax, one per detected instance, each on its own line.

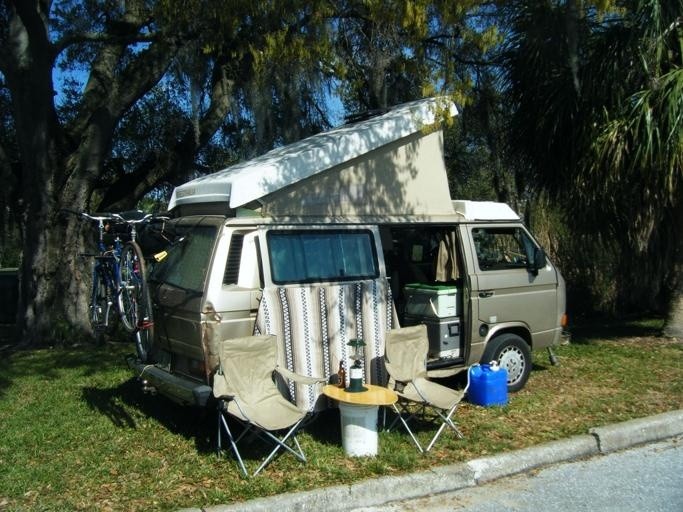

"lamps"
<box><xmin>345</xmin><ymin>339</ymin><xmax>370</xmax><ymax>392</ymax></box>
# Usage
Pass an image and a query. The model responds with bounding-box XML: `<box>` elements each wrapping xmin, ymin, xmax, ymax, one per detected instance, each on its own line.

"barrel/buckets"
<box><xmin>469</xmin><ymin>359</ymin><xmax>508</xmax><ymax>407</ymax></box>
<box><xmin>340</xmin><ymin>403</ymin><xmax>379</xmax><ymax>460</ymax></box>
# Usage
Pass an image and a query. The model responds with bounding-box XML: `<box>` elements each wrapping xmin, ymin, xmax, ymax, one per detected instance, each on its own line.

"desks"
<box><xmin>321</xmin><ymin>383</ymin><xmax>399</xmax><ymax>459</ymax></box>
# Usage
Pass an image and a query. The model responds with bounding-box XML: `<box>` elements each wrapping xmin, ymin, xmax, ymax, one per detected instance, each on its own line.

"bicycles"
<box><xmin>81</xmin><ymin>207</ymin><xmax>169</xmax><ymax>363</ymax></box>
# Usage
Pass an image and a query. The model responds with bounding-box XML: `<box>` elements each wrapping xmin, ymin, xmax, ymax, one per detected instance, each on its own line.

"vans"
<box><xmin>128</xmin><ymin>94</ymin><xmax>568</xmax><ymax>455</ymax></box>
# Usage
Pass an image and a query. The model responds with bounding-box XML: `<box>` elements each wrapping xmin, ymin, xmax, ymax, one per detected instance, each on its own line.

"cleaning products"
<box><xmin>337</xmin><ymin>360</ymin><xmax>346</xmax><ymax>387</ymax></box>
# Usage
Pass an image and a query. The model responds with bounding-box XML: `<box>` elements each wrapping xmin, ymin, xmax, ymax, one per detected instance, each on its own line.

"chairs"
<box><xmin>210</xmin><ymin>335</ymin><xmax>326</xmax><ymax>478</ymax></box>
<box><xmin>382</xmin><ymin>325</ymin><xmax>466</xmax><ymax>453</ymax></box>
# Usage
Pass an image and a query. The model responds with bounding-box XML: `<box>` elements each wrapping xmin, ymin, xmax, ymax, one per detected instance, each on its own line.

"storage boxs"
<box><xmin>406</xmin><ymin>312</ymin><xmax>463</xmax><ymax>357</ymax></box>
<box><xmin>404</xmin><ymin>283</ymin><xmax>462</xmax><ymax>319</ymax></box>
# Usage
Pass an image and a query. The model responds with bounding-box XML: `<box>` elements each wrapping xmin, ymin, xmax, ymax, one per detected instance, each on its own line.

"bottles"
<box><xmin>349</xmin><ymin>359</ymin><xmax>363</xmax><ymax>388</ymax></box>
<box><xmin>337</xmin><ymin>361</ymin><xmax>347</xmax><ymax>389</ymax></box>
